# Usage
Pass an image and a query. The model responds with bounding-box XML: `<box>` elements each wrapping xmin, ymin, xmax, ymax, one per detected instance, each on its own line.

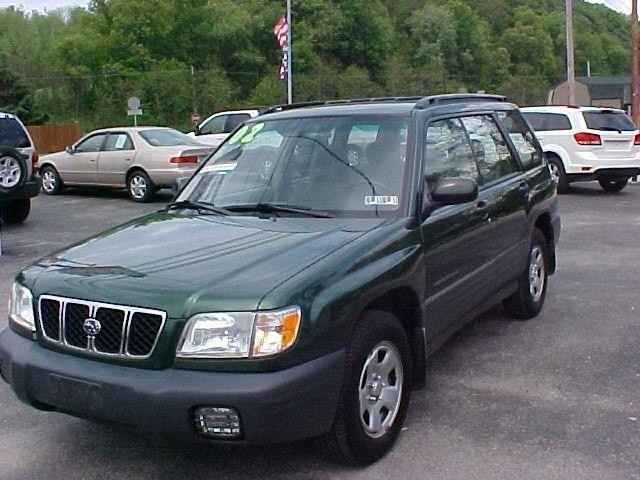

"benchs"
<box><xmin>273</xmin><ymin>14</ymin><xmax>289</xmax><ymax>81</ymax></box>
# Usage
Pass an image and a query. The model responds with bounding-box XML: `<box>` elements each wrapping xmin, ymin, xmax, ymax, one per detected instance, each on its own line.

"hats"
<box><xmin>39</xmin><ymin>110</ymin><xmax>261</xmax><ymax>208</ymax></box>
<box><xmin>516</xmin><ymin>104</ymin><xmax>640</xmax><ymax>194</ymax></box>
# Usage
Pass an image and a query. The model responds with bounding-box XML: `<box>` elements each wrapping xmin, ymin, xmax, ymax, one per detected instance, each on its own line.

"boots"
<box><xmin>0</xmin><ymin>110</ymin><xmax>42</xmax><ymax>230</ymax></box>
<box><xmin>0</xmin><ymin>94</ymin><xmax>562</xmax><ymax>467</ymax></box>
